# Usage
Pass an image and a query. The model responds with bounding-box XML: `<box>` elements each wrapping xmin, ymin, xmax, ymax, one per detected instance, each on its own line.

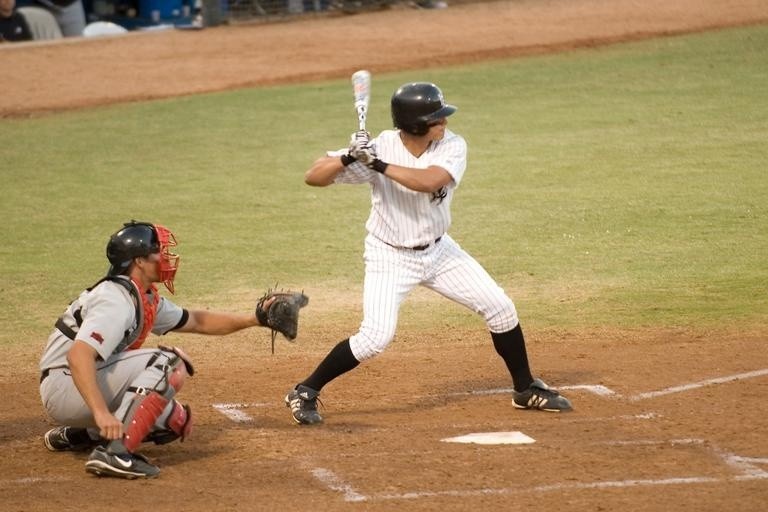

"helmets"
<box><xmin>106</xmin><ymin>219</ymin><xmax>179</xmax><ymax>295</ymax></box>
<box><xmin>391</xmin><ymin>82</ymin><xmax>457</xmax><ymax>137</ymax></box>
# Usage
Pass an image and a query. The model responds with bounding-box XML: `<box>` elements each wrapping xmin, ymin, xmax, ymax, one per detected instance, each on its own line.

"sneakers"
<box><xmin>512</xmin><ymin>378</ymin><xmax>571</xmax><ymax>412</ymax></box>
<box><xmin>85</xmin><ymin>445</ymin><xmax>160</xmax><ymax>480</ymax></box>
<box><xmin>284</xmin><ymin>382</ymin><xmax>324</xmax><ymax>425</ymax></box>
<box><xmin>44</xmin><ymin>424</ymin><xmax>95</xmax><ymax>453</ymax></box>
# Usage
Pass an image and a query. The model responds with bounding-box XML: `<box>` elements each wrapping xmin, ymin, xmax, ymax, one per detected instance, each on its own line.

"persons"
<box><xmin>0</xmin><ymin>0</ymin><xmax>65</xmax><ymax>47</ymax></box>
<box><xmin>38</xmin><ymin>221</ymin><xmax>310</xmax><ymax>478</ymax></box>
<box><xmin>284</xmin><ymin>79</ymin><xmax>575</xmax><ymax>426</ymax></box>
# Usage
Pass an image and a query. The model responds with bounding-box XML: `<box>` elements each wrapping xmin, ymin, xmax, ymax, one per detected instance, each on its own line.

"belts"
<box><xmin>40</xmin><ymin>369</ymin><xmax>49</xmax><ymax>383</ymax></box>
<box><xmin>413</xmin><ymin>238</ymin><xmax>441</xmax><ymax>251</ymax></box>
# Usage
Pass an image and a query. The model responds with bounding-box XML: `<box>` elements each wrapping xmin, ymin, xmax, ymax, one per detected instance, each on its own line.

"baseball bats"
<box><xmin>352</xmin><ymin>69</ymin><xmax>371</xmax><ymax>163</ymax></box>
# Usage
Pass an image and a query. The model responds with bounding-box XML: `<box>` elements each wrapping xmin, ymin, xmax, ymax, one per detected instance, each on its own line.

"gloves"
<box><xmin>340</xmin><ymin>132</ymin><xmax>387</xmax><ymax>174</ymax></box>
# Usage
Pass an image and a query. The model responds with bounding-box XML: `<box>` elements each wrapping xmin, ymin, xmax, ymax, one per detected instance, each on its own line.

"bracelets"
<box><xmin>340</xmin><ymin>155</ymin><xmax>357</xmax><ymax>168</ymax></box>
<box><xmin>372</xmin><ymin>159</ymin><xmax>389</xmax><ymax>174</ymax></box>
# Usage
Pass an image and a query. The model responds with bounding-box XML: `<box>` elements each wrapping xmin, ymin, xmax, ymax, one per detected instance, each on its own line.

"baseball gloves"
<box><xmin>255</xmin><ymin>290</ymin><xmax>308</xmax><ymax>342</ymax></box>
